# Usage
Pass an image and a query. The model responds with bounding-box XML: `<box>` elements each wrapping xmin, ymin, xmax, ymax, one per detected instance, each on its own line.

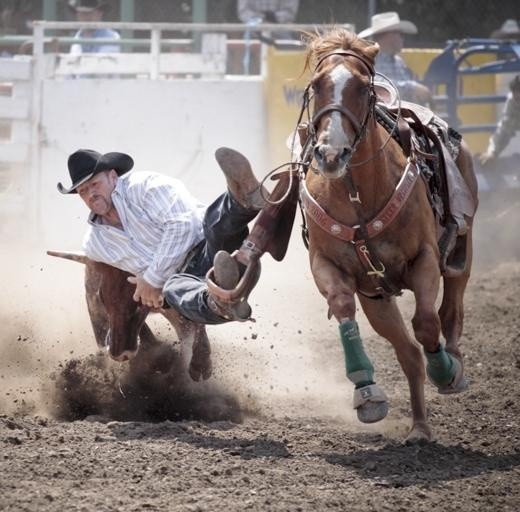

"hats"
<box><xmin>56</xmin><ymin>148</ymin><xmax>134</xmax><ymax>195</ymax></box>
<box><xmin>356</xmin><ymin>9</ymin><xmax>420</xmax><ymax>40</ymax></box>
<box><xmin>66</xmin><ymin>0</ymin><xmax>114</xmax><ymax>18</ymax></box>
<box><xmin>488</xmin><ymin>17</ymin><xmax>520</xmax><ymax>40</ymax></box>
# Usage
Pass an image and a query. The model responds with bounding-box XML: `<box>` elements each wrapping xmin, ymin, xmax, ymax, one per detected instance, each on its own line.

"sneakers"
<box><xmin>205</xmin><ymin>249</ymin><xmax>252</xmax><ymax>322</ymax></box>
<box><xmin>214</xmin><ymin>146</ymin><xmax>271</xmax><ymax>211</ymax></box>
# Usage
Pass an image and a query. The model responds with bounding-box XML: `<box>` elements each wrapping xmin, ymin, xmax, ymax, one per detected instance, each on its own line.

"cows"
<box><xmin>46</xmin><ymin>250</ymin><xmax>213</xmax><ymax>381</ymax></box>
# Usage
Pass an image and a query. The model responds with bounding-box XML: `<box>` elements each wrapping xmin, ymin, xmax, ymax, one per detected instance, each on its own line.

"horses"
<box><xmin>296</xmin><ymin>20</ymin><xmax>479</xmax><ymax>446</ymax></box>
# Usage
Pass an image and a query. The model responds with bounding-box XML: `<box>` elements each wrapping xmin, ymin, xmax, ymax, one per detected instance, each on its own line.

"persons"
<box><xmin>357</xmin><ymin>8</ymin><xmax>435</xmax><ymax>107</ymax></box>
<box><xmin>474</xmin><ymin>74</ymin><xmax>519</xmax><ymax>165</ymax></box>
<box><xmin>485</xmin><ymin>19</ymin><xmax>519</xmax><ymax>61</ymax></box>
<box><xmin>54</xmin><ymin>146</ymin><xmax>271</xmax><ymax>325</ymax></box>
<box><xmin>236</xmin><ymin>0</ymin><xmax>301</xmax><ymax>40</ymax></box>
<box><xmin>67</xmin><ymin>0</ymin><xmax>122</xmax><ymax>56</ymax></box>
